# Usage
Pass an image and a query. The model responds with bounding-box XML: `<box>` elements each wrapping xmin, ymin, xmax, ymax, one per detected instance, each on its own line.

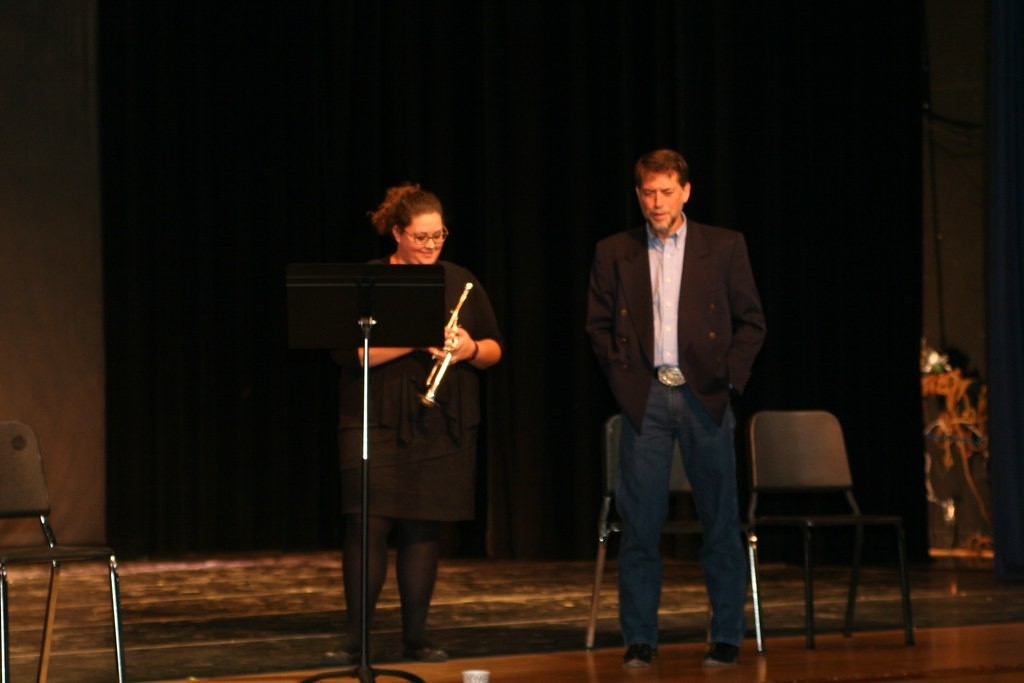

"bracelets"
<box><xmin>466</xmin><ymin>340</ymin><xmax>479</xmax><ymax>364</ymax></box>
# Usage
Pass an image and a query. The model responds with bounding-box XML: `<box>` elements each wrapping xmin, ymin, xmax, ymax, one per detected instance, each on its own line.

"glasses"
<box><xmin>398</xmin><ymin>224</ymin><xmax>449</xmax><ymax>245</ymax></box>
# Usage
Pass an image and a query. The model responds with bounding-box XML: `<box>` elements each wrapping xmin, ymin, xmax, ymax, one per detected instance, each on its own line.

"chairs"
<box><xmin>0</xmin><ymin>421</ymin><xmax>127</xmax><ymax>683</ymax></box>
<box><xmin>586</xmin><ymin>414</ymin><xmax>719</xmax><ymax>646</ymax></box>
<box><xmin>749</xmin><ymin>409</ymin><xmax>918</xmax><ymax>651</ymax></box>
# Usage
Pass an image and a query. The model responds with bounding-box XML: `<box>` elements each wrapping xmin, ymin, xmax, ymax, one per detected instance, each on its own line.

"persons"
<box><xmin>582</xmin><ymin>149</ymin><xmax>768</xmax><ymax>668</ymax></box>
<box><xmin>320</xmin><ymin>185</ymin><xmax>504</xmax><ymax>666</ymax></box>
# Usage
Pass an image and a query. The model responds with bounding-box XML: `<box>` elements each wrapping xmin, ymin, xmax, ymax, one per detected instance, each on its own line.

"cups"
<box><xmin>462</xmin><ymin>670</ymin><xmax>490</xmax><ymax>683</ymax></box>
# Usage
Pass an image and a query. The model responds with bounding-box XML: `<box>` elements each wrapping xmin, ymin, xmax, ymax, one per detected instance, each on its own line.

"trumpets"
<box><xmin>418</xmin><ymin>282</ymin><xmax>475</xmax><ymax>410</ymax></box>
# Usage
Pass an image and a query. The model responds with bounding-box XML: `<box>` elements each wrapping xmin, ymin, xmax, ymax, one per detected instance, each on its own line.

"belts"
<box><xmin>648</xmin><ymin>365</ymin><xmax>685</xmax><ymax>388</ymax></box>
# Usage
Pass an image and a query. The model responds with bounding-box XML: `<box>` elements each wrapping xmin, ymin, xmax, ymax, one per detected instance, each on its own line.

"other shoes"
<box><xmin>704</xmin><ymin>641</ymin><xmax>742</xmax><ymax>665</ymax></box>
<box><xmin>624</xmin><ymin>642</ymin><xmax>659</xmax><ymax>668</ymax></box>
<box><xmin>321</xmin><ymin>647</ymin><xmax>373</xmax><ymax>666</ymax></box>
<box><xmin>404</xmin><ymin>646</ymin><xmax>446</xmax><ymax>662</ymax></box>
